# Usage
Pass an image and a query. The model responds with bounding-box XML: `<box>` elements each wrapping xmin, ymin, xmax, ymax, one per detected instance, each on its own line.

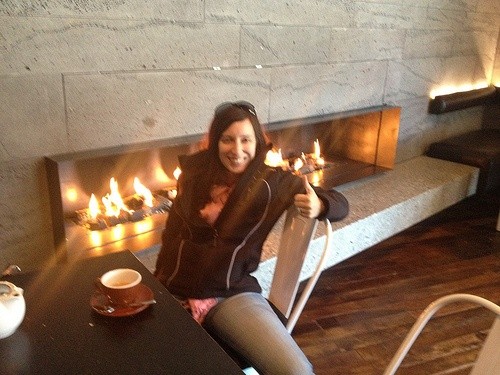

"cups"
<box><xmin>95</xmin><ymin>269</ymin><xmax>142</xmax><ymax>307</ymax></box>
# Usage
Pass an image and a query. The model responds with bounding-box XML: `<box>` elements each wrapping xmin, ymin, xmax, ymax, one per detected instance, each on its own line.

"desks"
<box><xmin>0</xmin><ymin>248</ymin><xmax>246</xmax><ymax>375</ymax></box>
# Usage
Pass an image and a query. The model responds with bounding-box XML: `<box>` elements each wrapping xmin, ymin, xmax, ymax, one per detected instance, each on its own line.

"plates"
<box><xmin>90</xmin><ymin>283</ymin><xmax>154</xmax><ymax>317</ymax></box>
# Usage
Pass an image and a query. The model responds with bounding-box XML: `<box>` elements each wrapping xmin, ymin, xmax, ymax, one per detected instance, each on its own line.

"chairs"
<box><xmin>383</xmin><ymin>293</ymin><xmax>500</xmax><ymax>375</ymax></box>
<box><xmin>206</xmin><ymin>200</ymin><xmax>333</xmax><ymax>375</ymax></box>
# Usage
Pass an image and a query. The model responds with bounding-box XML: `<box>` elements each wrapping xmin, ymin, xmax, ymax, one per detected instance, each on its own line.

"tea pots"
<box><xmin>0</xmin><ymin>281</ymin><xmax>26</xmax><ymax>339</ymax></box>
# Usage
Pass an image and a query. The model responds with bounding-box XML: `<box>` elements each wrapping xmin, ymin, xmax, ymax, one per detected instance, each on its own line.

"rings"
<box><xmin>297</xmin><ymin>208</ymin><xmax>301</xmax><ymax>213</ymax></box>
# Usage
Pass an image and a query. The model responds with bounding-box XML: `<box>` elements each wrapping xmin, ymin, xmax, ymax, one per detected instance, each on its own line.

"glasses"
<box><xmin>214</xmin><ymin>100</ymin><xmax>256</xmax><ymax>118</ymax></box>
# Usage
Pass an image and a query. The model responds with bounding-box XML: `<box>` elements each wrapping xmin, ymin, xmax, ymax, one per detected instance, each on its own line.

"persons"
<box><xmin>153</xmin><ymin>101</ymin><xmax>349</xmax><ymax>375</ymax></box>
